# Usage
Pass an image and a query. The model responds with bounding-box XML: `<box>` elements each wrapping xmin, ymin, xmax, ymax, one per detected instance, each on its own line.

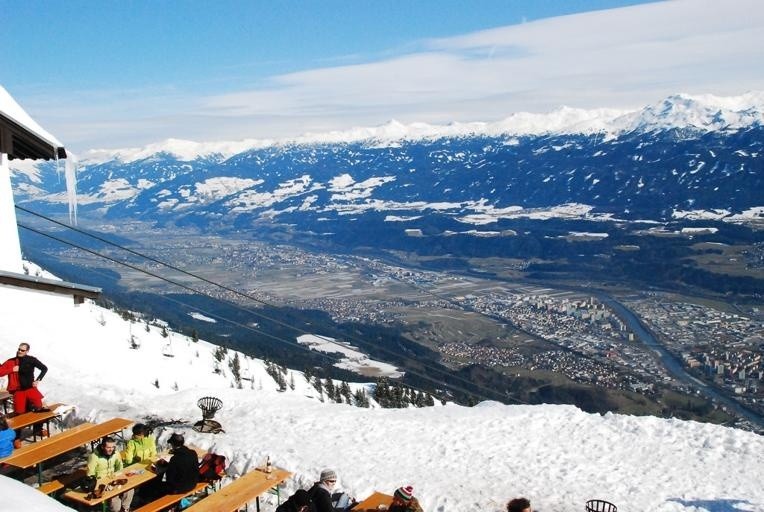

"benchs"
<box><xmin>0</xmin><ymin>388</ymin><xmax>225</xmax><ymax>512</ymax></box>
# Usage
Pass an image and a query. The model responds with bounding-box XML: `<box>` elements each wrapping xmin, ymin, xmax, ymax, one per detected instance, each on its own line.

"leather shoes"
<box><xmin>13</xmin><ymin>438</ymin><xmax>20</xmax><ymax>448</ymax></box>
<box><xmin>38</xmin><ymin>428</ymin><xmax>46</xmax><ymax>436</ymax></box>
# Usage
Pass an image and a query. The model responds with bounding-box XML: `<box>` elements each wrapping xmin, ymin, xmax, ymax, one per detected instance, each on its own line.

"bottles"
<box><xmin>266</xmin><ymin>456</ymin><xmax>272</xmax><ymax>474</ymax></box>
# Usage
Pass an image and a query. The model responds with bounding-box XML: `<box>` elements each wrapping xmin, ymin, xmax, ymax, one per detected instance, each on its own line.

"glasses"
<box><xmin>18</xmin><ymin>349</ymin><xmax>27</xmax><ymax>352</ymax></box>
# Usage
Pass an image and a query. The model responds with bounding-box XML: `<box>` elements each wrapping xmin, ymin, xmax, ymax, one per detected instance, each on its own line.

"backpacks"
<box><xmin>198</xmin><ymin>453</ymin><xmax>226</xmax><ymax>482</ymax></box>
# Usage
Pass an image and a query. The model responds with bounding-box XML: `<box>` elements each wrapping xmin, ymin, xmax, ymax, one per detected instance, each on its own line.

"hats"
<box><xmin>167</xmin><ymin>434</ymin><xmax>183</xmax><ymax>445</ymax></box>
<box><xmin>320</xmin><ymin>469</ymin><xmax>336</xmax><ymax>481</ymax></box>
<box><xmin>393</xmin><ymin>486</ymin><xmax>412</xmax><ymax>504</ymax></box>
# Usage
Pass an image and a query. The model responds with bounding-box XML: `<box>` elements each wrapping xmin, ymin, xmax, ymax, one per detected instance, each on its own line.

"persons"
<box><xmin>507</xmin><ymin>498</ymin><xmax>531</xmax><ymax>512</ymax></box>
<box><xmin>87</xmin><ymin>437</ymin><xmax>135</xmax><ymax>512</ymax></box>
<box><xmin>156</xmin><ymin>434</ymin><xmax>198</xmax><ymax>495</ymax></box>
<box><xmin>276</xmin><ymin>489</ymin><xmax>312</xmax><ymax>512</ymax></box>
<box><xmin>308</xmin><ymin>469</ymin><xmax>349</xmax><ymax>512</ymax></box>
<box><xmin>0</xmin><ymin>343</ymin><xmax>48</xmax><ymax>449</ymax></box>
<box><xmin>387</xmin><ymin>486</ymin><xmax>423</xmax><ymax>512</ymax></box>
<box><xmin>0</xmin><ymin>413</ymin><xmax>17</xmax><ymax>459</ymax></box>
<box><xmin>125</xmin><ymin>424</ymin><xmax>157</xmax><ymax>466</ymax></box>
